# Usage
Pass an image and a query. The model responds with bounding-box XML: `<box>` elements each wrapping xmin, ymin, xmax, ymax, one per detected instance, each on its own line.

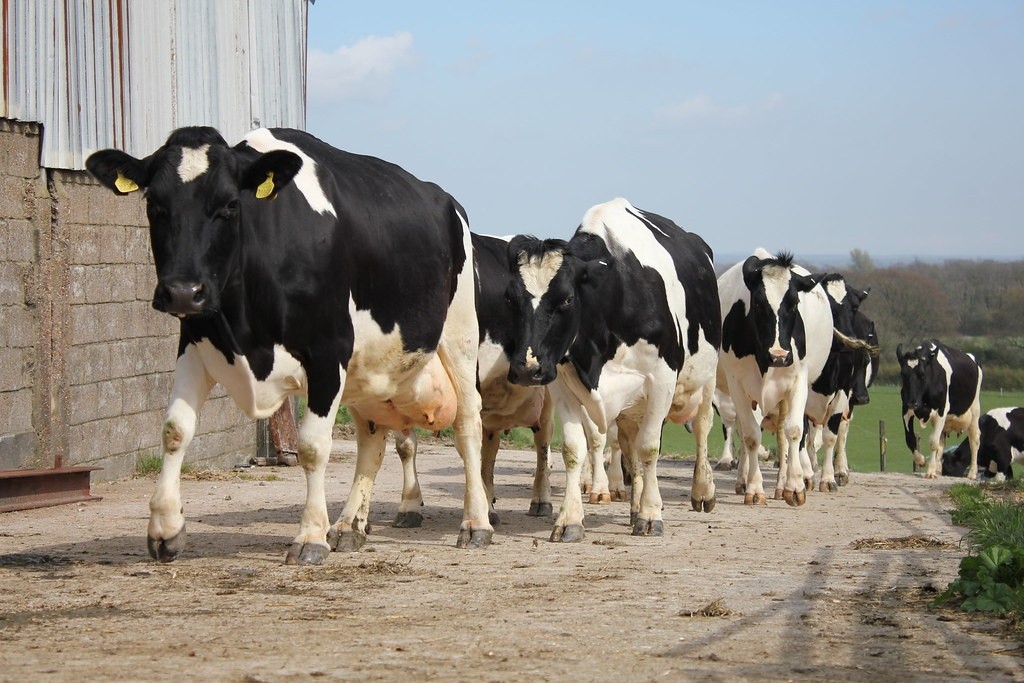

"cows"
<box><xmin>896</xmin><ymin>338</ymin><xmax>1024</xmax><ymax>484</ymax></box>
<box><xmin>85</xmin><ymin>125</ymin><xmax>879</xmax><ymax>566</ymax></box>
